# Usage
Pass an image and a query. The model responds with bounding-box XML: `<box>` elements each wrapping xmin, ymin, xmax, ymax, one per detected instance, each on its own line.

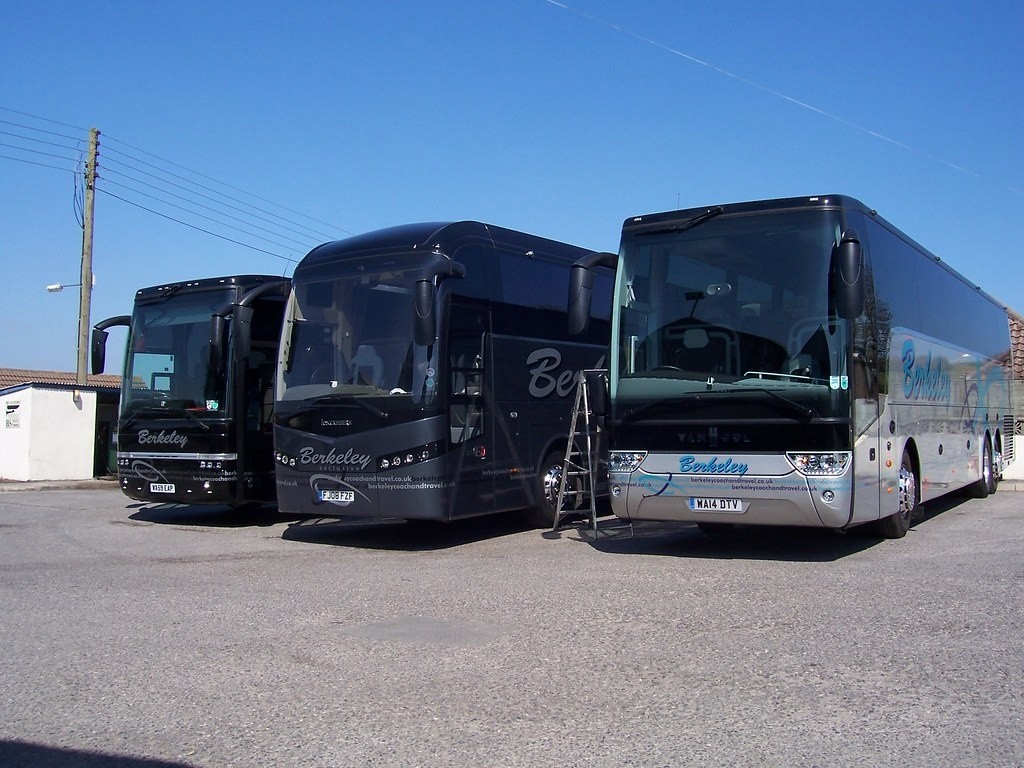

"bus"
<box><xmin>90</xmin><ymin>276</ymin><xmax>420</xmax><ymax>518</ymax></box>
<box><xmin>207</xmin><ymin>219</ymin><xmax>652</xmax><ymax>531</ymax></box>
<box><xmin>566</xmin><ymin>193</ymin><xmax>1016</xmax><ymax>538</ymax></box>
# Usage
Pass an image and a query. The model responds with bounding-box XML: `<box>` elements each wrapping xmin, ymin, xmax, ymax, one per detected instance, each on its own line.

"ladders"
<box><xmin>553</xmin><ymin>367</ymin><xmax>636</xmax><ymax>540</ymax></box>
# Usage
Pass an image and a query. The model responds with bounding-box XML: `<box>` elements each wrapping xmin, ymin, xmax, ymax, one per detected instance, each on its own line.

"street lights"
<box><xmin>45</xmin><ymin>283</ymin><xmax>95</xmax><ymax>385</ymax></box>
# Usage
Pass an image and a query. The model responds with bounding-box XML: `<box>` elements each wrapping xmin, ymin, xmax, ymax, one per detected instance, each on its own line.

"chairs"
<box><xmin>346</xmin><ymin>346</ymin><xmax>384</xmax><ymax>386</ymax></box>
